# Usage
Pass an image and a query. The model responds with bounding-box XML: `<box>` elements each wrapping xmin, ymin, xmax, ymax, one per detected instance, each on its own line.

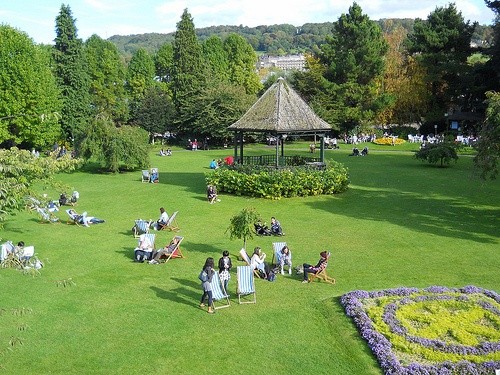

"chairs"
<box><xmin>206</xmin><ymin>185</ymin><xmax>217</xmax><ymax>201</ymax></box>
<box><xmin>38</xmin><ymin>208</ymin><xmax>62</xmax><ymax>226</ymax></box>
<box><xmin>133</xmin><ymin>219</ymin><xmax>152</xmax><ymax>240</ymax></box>
<box><xmin>3</xmin><ymin>241</ymin><xmax>24</xmax><ymax>269</ymax></box>
<box><xmin>271</xmin><ymin>241</ymin><xmax>291</xmax><ymax>271</ymax></box>
<box><xmin>307</xmin><ymin>251</ymin><xmax>336</xmax><ymax>284</ymax></box>
<box><xmin>344</xmin><ymin>132</ymin><xmax>399</xmax><ymax>144</ymax></box>
<box><xmin>205</xmin><ymin>269</ymin><xmax>231</xmax><ymax>310</ymax></box>
<box><xmin>408</xmin><ymin>134</ymin><xmax>471</xmax><ymax>147</ymax></box>
<box><xmin>134</xmin><ymin>233</ymin><xmax>158</xmax><ymax>258</ymax></box>
<box><xmin>151</xmin><ymin>168</ymin><xmax>159</xmax><ymax>182</ymax></box>
<box><xmin>142</xmin><ymin>170</ymin><xmax>150</xmax><ymax>183</ymax></box>
<box><xmin>156</xmin><ymin>234</ymin><xmax>186</xmax><ymax>264</ymax></box>
<box><xmin>239</xmin><ymin>248</ymin><xmax>262</xmax><ymax>279</ymax></box>
<box><xmin>159</xmin><ymin>210</ymin><xmax>180</xmax><ymax>231</ymax></box>
<box><xmin>236</xmin><ymin>265</ymin><xmax>257</xmax><ymax>304</ymax></box>
<box><xmin>66</xmin><ymin>208</ymin><xmax>88</xmax><ymax>228</ymax></box>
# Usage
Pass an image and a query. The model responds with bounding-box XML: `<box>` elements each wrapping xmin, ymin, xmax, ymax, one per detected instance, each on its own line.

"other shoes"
<box><xmin>302</xmin><ymin>280</ymin><xmax>308</xmax><ymax>283</ymax></box>
<box><xmin>281</xmin><ymin>270</ymin><xmax>284</xmax><ymax>275</ymax></box>
<box><xmin>148</xmin><ymin>259</ymin><xmax>159</xmax><ymax>264</ymax></box>
<box><xmin>288</xmin><ymin>269</ymin><xmax>292</xmax><ymax>274</ymax></box>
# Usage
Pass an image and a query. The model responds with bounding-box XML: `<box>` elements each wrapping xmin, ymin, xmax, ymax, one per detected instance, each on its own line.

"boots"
<box><xmin>199</xmin><ymin>303</ymin><xmax>206</xmax><ymax>307</ymax></box>
<box><xmin>208</xmin><ymin>306</ymin><xmax>215</xmax><ymax>314</ymax></box>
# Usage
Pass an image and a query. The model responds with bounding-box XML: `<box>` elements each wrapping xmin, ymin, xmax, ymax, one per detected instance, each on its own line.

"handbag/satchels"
<box><xmin>268</xmin><ymin>269</ymin><xmax>275</xmax><ymax>281</ymax></box>
<box><xmin>199</xmin><ymin>271</ymin><xmax>208</xmax><ymax>283</ymax></box>
<box><xmin>259</xmin><ymin>269</ymin><xmax>265</xmax><ymax>278</ymax></box>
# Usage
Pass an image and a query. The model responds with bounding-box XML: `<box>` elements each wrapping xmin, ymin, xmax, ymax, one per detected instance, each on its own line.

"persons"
<box><xmin>200</xmin><ymin>257</ymin><xmax>215</xmax><ymax>314</ymax></box>
<box><xmin>13</xmin><ymin>131</ymin><xmax>480</xmax><ymax>295</ymax></box>
